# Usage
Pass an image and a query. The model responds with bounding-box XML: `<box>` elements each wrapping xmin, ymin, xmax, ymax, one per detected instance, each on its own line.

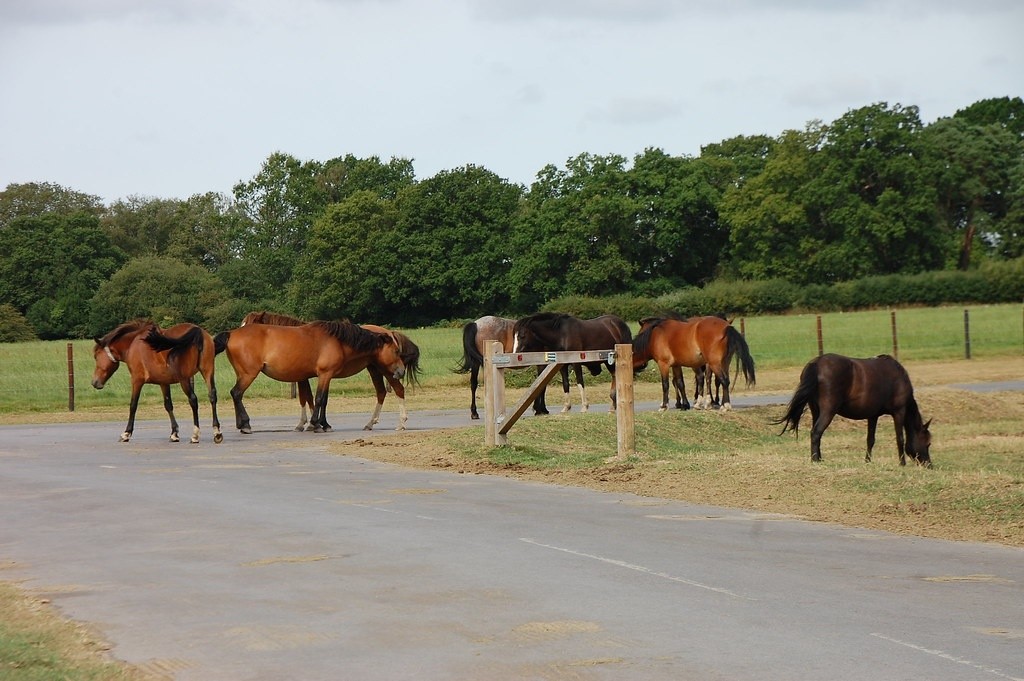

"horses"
<box><xmin>764</xmin><ymin>352</ymin><xmax>933</xmax><ymax>470</ymax></box>
<box><xmin>90</xmin><ymin>309</ymin><xmax>756</xmax><ymax>442</ymax></box>
